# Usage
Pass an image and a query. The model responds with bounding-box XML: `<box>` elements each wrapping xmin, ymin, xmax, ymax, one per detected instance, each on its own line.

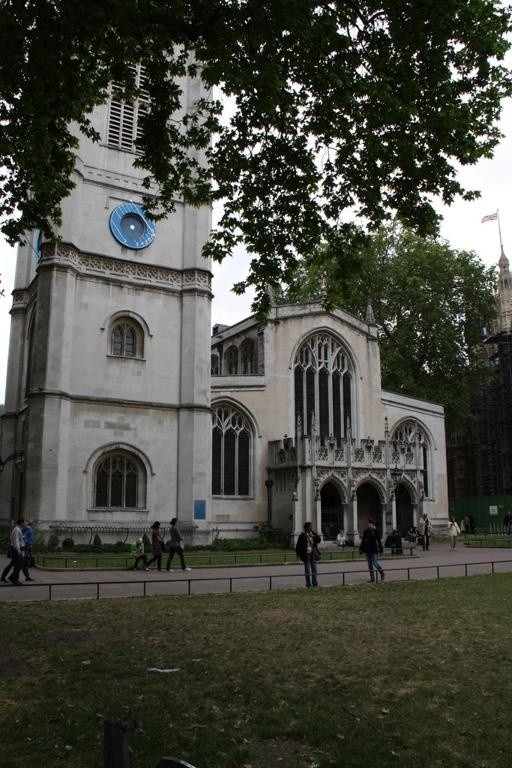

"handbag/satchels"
<box><xmin>7</xmin><ymin>547</ymin><xmax>19</xmax><ymax>557</ymax></box>
<box><xmin>24</xmin><ymin>555</ymin><xmax>36</xmax><ymax>568</ymax></box>
<box><xmin>312</xmin><ymin>546</ymin><xmax>321</xmax><ymax>560</ymax></box>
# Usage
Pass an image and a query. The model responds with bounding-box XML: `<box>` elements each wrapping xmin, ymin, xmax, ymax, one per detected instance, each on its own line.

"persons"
<box><xmin>164</xmin><ymin>517</ymin><xmax>192</xmax><ymax>572</ymax></box>
<box><xmin>295</xmin><ymin>521</ymin><xmax>321</xmax><ymax>587</ymax></box>
<box><xmin>9</xmin><ymin>518</ymin><xmax>29</xmax><ymax>585</ymax></box>
<box><xmin>384</xmin><ymin>526</ymin><xmax>423</xmax><ymax>556</ymax></box>
<box><xmin>458</xmin><ymin>513</ymin><xmax>475</xmax><ymax>535</ymax></box>
<box><xmin>336</xmin><ymin>528</ymin><xmax>346</xmax><ymax>546</ymax></box>
<box><xmin>503</xmin><ymin>511</ymin><xmax>511</xmax><ymax>535</ymax></box>
<box><xmin>1</xmin><ymin>520</ymin><xmax>36</xmax><ymax>581</ymax></box>
<box><xmin>446</xmin><ymin>515</ymin><xmax>460</xmax><ymax>552</ymax></box>
<box><xmin>144</xmin><ymin>520</ymin><xmax>166</xmax><ymax>573</ymax></box>
<box><xmin>418</xmin><ymin>512</ymin><xmax>432</xmax><ymax>552</ymax></box>
<box><xmin>359</xmin><ymin>516</ymin><xmax>385</xmax><ymax>582</ymax></box>
<box><xmin>129</xmin><ymin>537</ymin><xmax>149</xmax><ymax>570</ymax></box>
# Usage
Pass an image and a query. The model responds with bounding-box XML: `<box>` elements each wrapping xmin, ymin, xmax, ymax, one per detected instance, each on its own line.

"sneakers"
<box><xmin>167</xmin><ymin>568</ymin><xmax>175</xmax><ymax>573</ymax></box>
<box><xmin>183</xmin><ymin>566</ymin><xmax>191</xmax><ymax>573</ymax></box>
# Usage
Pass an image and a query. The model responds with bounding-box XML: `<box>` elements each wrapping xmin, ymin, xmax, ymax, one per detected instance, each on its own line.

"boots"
<box><xmin>378</xmin><ymin>567</ymin><xmax>386</xmax><ymax>581</ymax></box>
<box><xmin>368</xmin><ymin>572</ymin><xmax>375</xmax><ymax>583</ymax></box>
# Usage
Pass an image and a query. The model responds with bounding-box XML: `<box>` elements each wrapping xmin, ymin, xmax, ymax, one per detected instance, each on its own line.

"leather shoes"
<box><xmin>2</xmin><ymin>577</ymin><xmax>36</xmax><ymax>584</ymax></box>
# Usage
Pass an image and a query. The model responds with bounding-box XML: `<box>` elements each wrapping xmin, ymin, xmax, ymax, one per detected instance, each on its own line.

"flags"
<box><xmin>480</xmin><ymin>212</ymin><xmax>497</xmax><ymax>223</ymax></box>
